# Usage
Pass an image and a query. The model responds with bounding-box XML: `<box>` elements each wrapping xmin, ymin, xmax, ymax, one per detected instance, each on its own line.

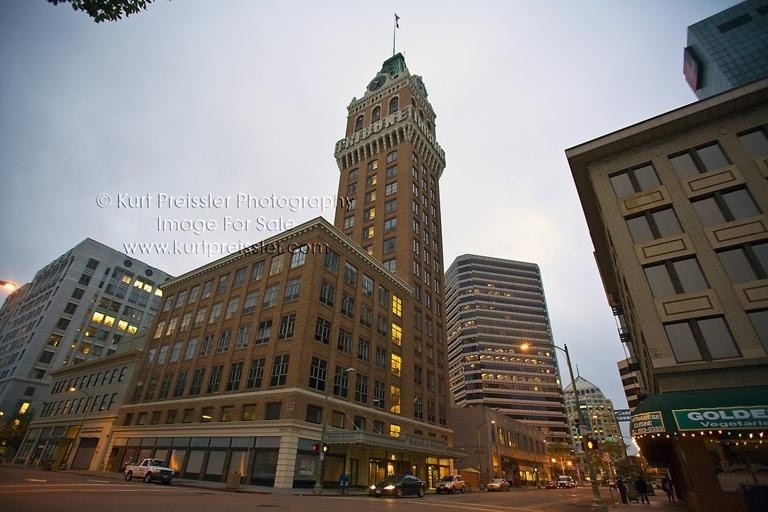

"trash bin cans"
<box><xmin>742</xmin><ymin>484</ymin><xmax>768</xmax><ymax>512</ymax></box>
<box><xmin>339</xmin><ymin>474</ymin><xmax>350</xmax><ymax>495</ymax></box>
<box><xmin>227</xmin><ymin>469</ymin><xmax>240</xmax><ymax>489</ymax></box>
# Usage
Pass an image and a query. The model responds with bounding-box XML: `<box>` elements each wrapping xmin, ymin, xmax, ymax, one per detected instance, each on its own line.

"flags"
<box><xmin>395</xmin><ymin>13</ymin><xmax>401</xmax><ymax>28</ymax></box>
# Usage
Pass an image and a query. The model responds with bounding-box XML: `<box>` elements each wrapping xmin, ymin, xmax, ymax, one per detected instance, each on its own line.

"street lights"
<box><xmin>619</xmin><ymin>436</ymin><xmax>649</xmax><ymax>480</ymax></box>
<box><xmin>60</xmin><ymin>387</ymin><xmax>92</xmax><ymax>470</ymax></box>
<box><xmin>519</xmin><ymin>340</ymin><xmax>608</xmax><ymax>512</ymax></box>
<box><xmin>476</xmin><ymin>420</ymin><xmax>496</xmax><ymax>489</ymax></box>
<box><xmin>313</xmin><ymin>366</ymin><xmax>355</xmax><ymax>496</ymax></box>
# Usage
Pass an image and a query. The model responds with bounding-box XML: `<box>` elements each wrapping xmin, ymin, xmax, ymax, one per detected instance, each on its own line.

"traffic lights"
<box><xmin>582</xmin><ymin>437</ymin><xmax>598</xmax><ymax>451</ymax></box>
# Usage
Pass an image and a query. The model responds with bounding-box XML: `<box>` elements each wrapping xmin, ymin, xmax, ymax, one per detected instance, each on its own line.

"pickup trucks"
<box><xmin>123</xmin><ymin>457</ymin><xmax>173</xmax><ymax>485</ymax></box>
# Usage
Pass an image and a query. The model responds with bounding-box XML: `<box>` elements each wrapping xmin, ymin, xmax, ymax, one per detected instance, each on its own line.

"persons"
<box><xmin>614</xmin><ymin>476</ymin><xmax>630</xmax><ymax>505</ymax></box>
<box><xmin>634</xmin><ymin>475</ymin><xmax>651</xmax><ymax>505</ymax></box>
<box><xmin>660</xmin><ymin>473</ymin><xmax>677</xmax><ymax>505</ymax></box>
<box><xmin>338</xmin><ymin>470</ymin><xmax>347</xmax><ymax>497</ymax></box>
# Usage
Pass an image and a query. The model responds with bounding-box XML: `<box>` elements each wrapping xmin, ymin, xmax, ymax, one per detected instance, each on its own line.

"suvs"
<box><xmin>436</xmin><ymin>474</ymin><xmax>465</xmax><ymax>493</ymax></box>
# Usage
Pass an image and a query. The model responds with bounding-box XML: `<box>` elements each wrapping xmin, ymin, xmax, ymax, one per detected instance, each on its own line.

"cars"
<box><xmin>487</xmin><ymin>478</ymin><xmax>510</xmax><ymax>491</ymax></box>
<box><xmin>546</xmin><ymin>474</ymin><xmax>575</xmax><ymax>488</ymax></box>
<box><xmin>367</xmin><ymin>473</ymin><xmax>428</xmax><ymax>498</ymax></box>
